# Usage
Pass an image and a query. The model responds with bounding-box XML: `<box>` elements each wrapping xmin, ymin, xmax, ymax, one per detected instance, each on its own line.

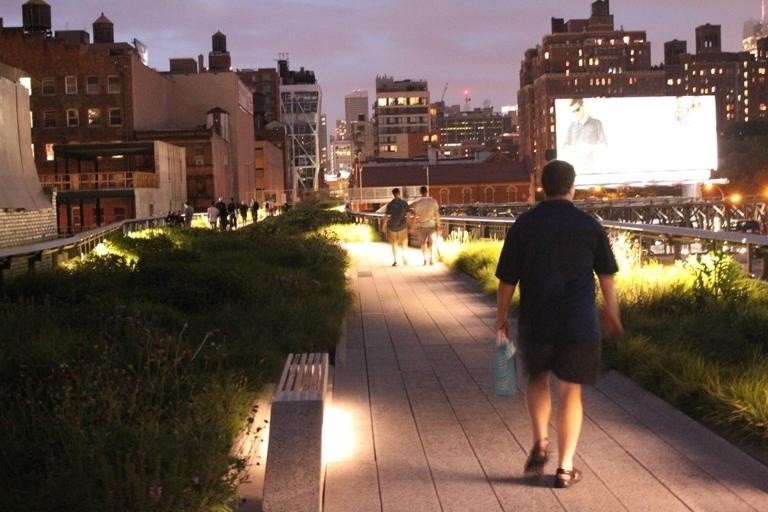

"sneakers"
<box><xmin>391</xmin><ymin>257</ymin><xmax>435</xmax><ymax>267</ymax></box>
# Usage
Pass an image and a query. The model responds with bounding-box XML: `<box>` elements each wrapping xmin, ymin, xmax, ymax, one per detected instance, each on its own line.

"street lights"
<box><xmin>422</xmin><ymin>164</ymin><xmax>429</xmax><ymax>200</ymax></box>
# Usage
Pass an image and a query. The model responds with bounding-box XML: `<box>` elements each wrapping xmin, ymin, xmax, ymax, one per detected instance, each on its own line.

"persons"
<box><xmin>384</xmin><ymin>188</ymin><xmax>414</xmax><ymax>266</ymax></box>
<box><xmin>562</xmin><ymin>97</ymin><xmax>605</xmax><ymax>144</ymax></box>
<box><xmin>262</xmin><ymin>197</ymin><xmax>292</xmax><ymax>219</ymax></box>
<box><xmin>205</xmin><ymin>196</ymin><xmax>239</xmax><ymax>231</ymax></box>
<box><xmin>239</xmin><ymin>200</ymin><xmax>248</xmax><ymax>228</ymax></box>
<box><xmin>493</xmin><ymin>160</ymin><xmax>626</xmax><ymax>490</ymax></box>
<box><xmin>653</xmin><ymin>96</ymin><xmax>719</xmax><ymax>181</ymax></box>
<box><xmin>165</xmin><ymin>200</ymin><xmax>194</xmax><ymax>229</ymax></box>
<box><xmin>249</xmin><ymin>197</ymin><xmax>259</xmax><ymax>223</ymax></box>
<box><xmin>409</xmin><ymin>185</ymin><xmax>442</xmax><ymax>266</ymax></box>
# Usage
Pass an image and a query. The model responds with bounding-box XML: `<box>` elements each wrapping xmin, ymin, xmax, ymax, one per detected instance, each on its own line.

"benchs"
<box><xmin>259</xmin><ymin>348</ymin><xmax>333</xmax><ymax>512</ymax></box>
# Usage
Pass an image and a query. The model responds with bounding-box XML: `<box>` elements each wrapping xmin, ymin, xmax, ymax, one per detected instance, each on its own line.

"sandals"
<box><xmin>523</xmin><ymin>436</ymin><xmax>550</xmax><ymax>482</ymax></box>
<box><xmin>554</xmin><ymin>466</ymin><xmax>583</xmax><ymax>490</ymax></box>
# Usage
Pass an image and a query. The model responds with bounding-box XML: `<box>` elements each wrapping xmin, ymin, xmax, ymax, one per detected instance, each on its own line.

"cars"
<box><xmin>648</xmin><ymin>242</ymin><xmax>675</xmax><ymax>256</ymax></box>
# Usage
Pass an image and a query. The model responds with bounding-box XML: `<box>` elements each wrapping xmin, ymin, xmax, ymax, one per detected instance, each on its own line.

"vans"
<box><xmin>723</xmin><ymin>219</ymin><xmax>761</xmax><ymax>238</ymax></box>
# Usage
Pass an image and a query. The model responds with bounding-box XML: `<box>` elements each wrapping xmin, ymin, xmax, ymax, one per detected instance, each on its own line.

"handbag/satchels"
<box><xmin>491</xmin><ymin>329</ymin><xmax>519</xmax><ymax>396</ymax></box>
